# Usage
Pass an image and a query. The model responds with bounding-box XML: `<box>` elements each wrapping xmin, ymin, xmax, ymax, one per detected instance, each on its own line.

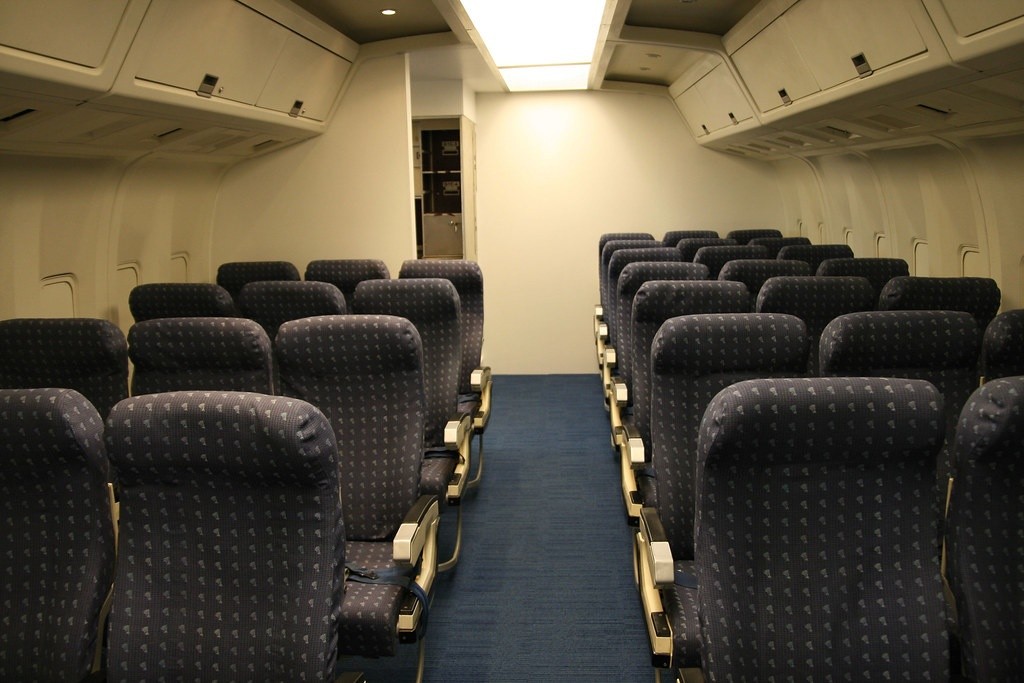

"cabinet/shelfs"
<box><xmin>720</xmin><ymin>0</ymin><xmax>1024</xmax><ymax>157</ymax></box>
<box><xmin>0</xmin><ymin>0</ymin><xmax>153</xmax><ymax>140</ymax></box>
<box><xmin>922</xmin><ymin>0</ymin><xmax>1024</xmax><ymax>87</ymax></box>
<box><xmin>668</xmin><ymin>52</ymin><xmax>793</xmax><ymax>160</ymax></box>
<box><xmin>0</xmin><ymin>0</ymin><xmax>360</xmax><ymax>162</ymax></box>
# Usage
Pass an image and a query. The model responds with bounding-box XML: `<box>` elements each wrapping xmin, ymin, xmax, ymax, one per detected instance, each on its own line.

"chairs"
<box><xmin>0</xmin><ymin>260</ymin><xmax>492</xmax><ymax>683</ymax></box>
<box><xmin>593</xmin><ymin>229</ymin><xmax>1024</xmax><ymax>683</ymax></box>
